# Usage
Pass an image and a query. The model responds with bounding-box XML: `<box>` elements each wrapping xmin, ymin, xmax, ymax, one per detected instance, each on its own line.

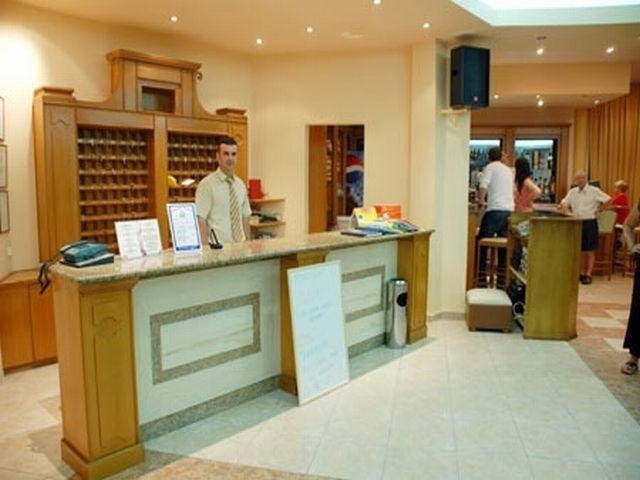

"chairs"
<box><xmin>591</xmin><ymin>206</ymin><xmax>640</xmax><ymax>280</ymax></box>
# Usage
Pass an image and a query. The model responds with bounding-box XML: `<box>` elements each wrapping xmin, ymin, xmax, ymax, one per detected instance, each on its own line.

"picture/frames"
<box><xmin>0</xmin><ymin>96</ymin><xmax>11</xmax><ymax>237</ymax></box>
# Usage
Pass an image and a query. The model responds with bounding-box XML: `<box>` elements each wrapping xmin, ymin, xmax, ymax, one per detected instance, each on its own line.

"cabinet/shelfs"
<box><xmin>249</xmin><ymin>196</ymin><xmax>286</xmax><ymax>231</ymax></box>
<box><xmin>1</xmin><ymin>283</ymin><xmax>56</xmax><ymax>372</ymax></box>
<box><xmin>509</xmin><ymin>233</ymin><xmax>528</xmax><ymax>336</ymax></box>
<box><xmin>161</xmin><ymin>115</ymin><xmax>250</xmax><ymax>251</ymax></box>
<box><xmin>33</xmin><ymin>102</ymin><xmax>163</xmax><ymax>257</ymax></box>
<box><xmin>310</xmin><ymin>124</ymin><xmax>346</xmax><ymax>233</ymax></box>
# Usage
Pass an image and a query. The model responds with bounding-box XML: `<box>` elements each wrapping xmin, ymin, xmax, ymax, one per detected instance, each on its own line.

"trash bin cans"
<box><xmin>385</xmin><ymin>277</ymin><xmax>408</xmax><ymax>348</ymax></box>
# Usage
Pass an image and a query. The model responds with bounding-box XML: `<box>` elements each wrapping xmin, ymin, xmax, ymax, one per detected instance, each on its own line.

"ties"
<box><xmin>224</xmin><ymin>177</ymin><xmax>243</xmax><ymax>244</ymax></box>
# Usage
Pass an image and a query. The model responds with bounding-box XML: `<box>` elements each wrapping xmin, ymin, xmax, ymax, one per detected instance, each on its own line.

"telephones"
<box><xmin>59</xmin><ymin>239</ymin><xmax>106</xmax><ymax>263</ymax></box>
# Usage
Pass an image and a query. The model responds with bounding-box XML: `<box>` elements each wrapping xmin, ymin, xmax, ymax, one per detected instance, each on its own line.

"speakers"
<box><xmin>450</xmin><ymin>45</ymin><xmax>490</xmax><ymax>111</ymax></box>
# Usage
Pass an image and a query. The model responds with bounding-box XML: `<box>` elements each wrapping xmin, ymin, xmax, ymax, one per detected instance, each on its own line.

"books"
<box><xmin>339</xmin><ymin>229</ymin><xmax>382</xmax><ymax>239</ymax></box>
<box><xmin>354</xmin><ymin>221</ymin><xmax>403</xmax><ymax>236</ymax></box>
<box><xmin>392</xmin><ymin>217</ymin><xmax>422</xmax><ymax>233</ymax></box>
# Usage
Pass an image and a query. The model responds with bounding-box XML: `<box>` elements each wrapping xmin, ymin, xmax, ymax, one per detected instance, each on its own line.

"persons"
<box><xmin>559</xmin><ymin>168</ymin><xmax>610</xmax><ymax>285</ymax></box>
<box><xmin>193</xmin><ymin>134</ymin><xmax>254</xmax><ymax>246</ymax></box>
<box><xmin>473</xmin><ymin>145</ymin><xmax>514</xmax><ymax>289</ymax></box>
<box><xmin>604</xmin><ymin>178</ymin><xmax>632</xmax><ymax>254</ymax></box>
<box><xmin>512</xmin><ymin>156</ymin><xmax>543</xmax><ymax>215</ymax></box>
<box><xmin>620</xmin><ymin>197</ymin><xmax>640</xmax><ymax>376</ymax></box>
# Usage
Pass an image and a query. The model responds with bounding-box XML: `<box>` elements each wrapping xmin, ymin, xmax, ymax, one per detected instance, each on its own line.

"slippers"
<box><xmin>579</xmin><ymin>271</ymin><xmax>592</xmax><ymax>285</ymax></box>
<box><xmin>620</xmin><ymin>356</ymin><xmax>638</xmax><ymax>374</ymax></box>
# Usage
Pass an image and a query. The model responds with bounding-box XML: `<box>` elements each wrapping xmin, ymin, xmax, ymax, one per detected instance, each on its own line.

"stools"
<box><xmin>476</xmin><ymin>235</ymin><xmax>509</xmax><ymax>290</ymax></box>
<box><xmin>467</xmin><ymin>288</ymin><xmax>514</xmax><ymax>334</ymax></box>
